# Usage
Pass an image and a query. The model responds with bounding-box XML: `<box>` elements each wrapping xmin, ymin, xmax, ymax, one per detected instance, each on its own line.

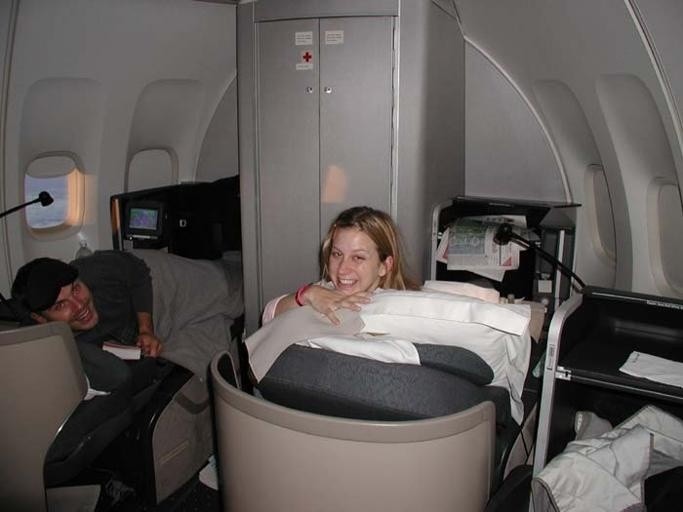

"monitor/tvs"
<box><xmin>124</xmin><ymin>202</ymin><xmax>164</xmax><ymax>236</ymax></box>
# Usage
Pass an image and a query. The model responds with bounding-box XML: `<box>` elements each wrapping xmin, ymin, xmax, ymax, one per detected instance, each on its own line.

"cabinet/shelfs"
<box><xmin>234</xmin><ymin>0</ymin><xmax>466</xmax><ymax>342</ymax></box>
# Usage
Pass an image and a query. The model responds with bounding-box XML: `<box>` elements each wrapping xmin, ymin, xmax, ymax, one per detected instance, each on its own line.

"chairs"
<box><xmin>0</xmin><ymin>249</ymin><xmax>248</xmax><ymax>512</ymax></box>
<box><xmin>208</xmin><ymin>278</ymin><xmax>554</xmax><ymax>512</ymax></box>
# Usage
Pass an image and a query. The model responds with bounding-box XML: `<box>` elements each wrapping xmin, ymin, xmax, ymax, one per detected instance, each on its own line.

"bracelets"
<box><xmin>295</xmin><ymin>283</ymin><xmax>309</xmax><ymax>306</ymax></box>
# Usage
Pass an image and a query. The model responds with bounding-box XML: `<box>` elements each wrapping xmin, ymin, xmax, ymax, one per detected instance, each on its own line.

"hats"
<box><xmin>12</xmin><ymin>258</ymin><xmax>79</xmax><ymax>312</ymax></box>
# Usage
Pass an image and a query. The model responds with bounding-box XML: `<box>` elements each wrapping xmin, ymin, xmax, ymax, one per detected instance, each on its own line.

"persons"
<box><xmin>261</xmin><ymin>206</ymin><xmax>420</xmax><ymax>326</ymax></box>
<box><xmin>10</xmin><ymin>248</ymin><xmax>164</xmax><ymax>391</ymax></box>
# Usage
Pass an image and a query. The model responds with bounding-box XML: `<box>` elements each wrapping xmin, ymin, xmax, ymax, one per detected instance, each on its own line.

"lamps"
<box><xmin>0</xmin><ymin>190</ymin><xmax>55</xmax><ymax>220</ymax></box>
<box><xmin>491</xmin><ymin>223</ymin><xmax>585</xmax><ymax>288</ymax></box>
